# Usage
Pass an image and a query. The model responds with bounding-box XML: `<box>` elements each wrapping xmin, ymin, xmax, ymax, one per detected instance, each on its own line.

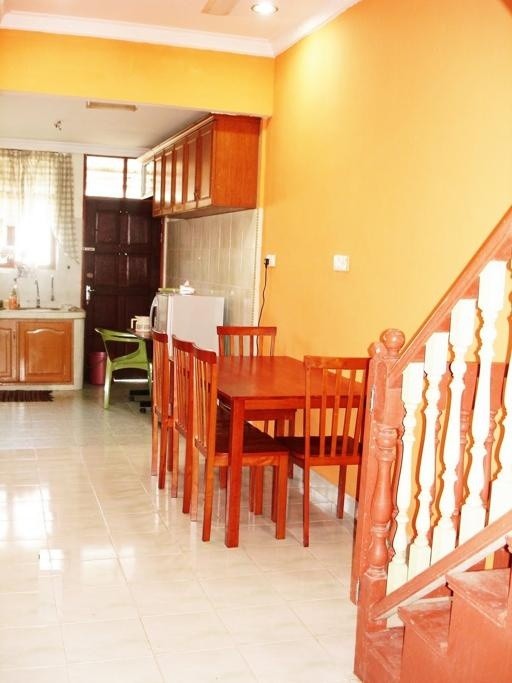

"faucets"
<box><xmin>34</xmin><ymin>279</ymin><xmax>39</xmax><ymax>297</ymax></box>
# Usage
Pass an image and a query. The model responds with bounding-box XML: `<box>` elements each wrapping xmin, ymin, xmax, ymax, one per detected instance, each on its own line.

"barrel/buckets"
<box><xmin>88</xmin><ymin>352</ymin><xmax>112</xmax><ymax>385</ymax></box>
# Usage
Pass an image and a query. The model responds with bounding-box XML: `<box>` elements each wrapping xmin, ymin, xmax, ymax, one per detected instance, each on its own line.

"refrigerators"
<box><xmin>149</xmin><ymin>294</ymin><xmax>225</xmax><ymax>426</ymax></box>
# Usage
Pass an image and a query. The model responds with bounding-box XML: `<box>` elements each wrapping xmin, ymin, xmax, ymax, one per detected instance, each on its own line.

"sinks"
<box><xmin>15</xmin><ymin>307</ymin><xmax>60</xmax><ymax>310</ymax></box>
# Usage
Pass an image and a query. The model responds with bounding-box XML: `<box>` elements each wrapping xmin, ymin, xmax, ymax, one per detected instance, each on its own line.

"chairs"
<box><xmin>269</xmin><ymin>353</ymin><xmax>372</xmax><ymax>547</ymax></box>
<box><xmin>169</xmin><ymin>333</ymin><xmax>263</xmax><ymax>517</ymax></box>
<box><xmin>188</xmin><ymin>342</ymin><xmax>291</xmax><ymax>543</ymax></box>
<box><xmin>215</xmin><ymin>327</ymin><xmax>280</xmax><ymax>358</ymax></box>
<box><xmin>150</xmin><ymin>329</ymin><xmax>231</xmax><ymax>491</ymax></box>
<box><xmin>96</xmin><ymin>327</ymin><xmax>152</xmax><ymax>410</ymax></box>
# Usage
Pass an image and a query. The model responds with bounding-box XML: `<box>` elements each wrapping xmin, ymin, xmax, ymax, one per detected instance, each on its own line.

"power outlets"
<box><xmin>266</xmin><ymin>255</ymin><xmax>276</xmax><ymax>267</ymax></box>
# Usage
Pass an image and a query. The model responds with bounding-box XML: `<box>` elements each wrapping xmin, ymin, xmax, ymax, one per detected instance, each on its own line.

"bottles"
<box><xmin>9</xmin><ymin>288</ymin><xmax>17</xmax><ymax>309</ymax></box>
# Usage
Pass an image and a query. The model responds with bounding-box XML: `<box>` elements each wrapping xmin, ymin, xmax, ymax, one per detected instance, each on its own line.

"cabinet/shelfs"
<box><xmin>174</xmin><ymin>130</ymin><xmax>197</xmax><ymax>210</ymax></box>
<box><xmin>196</xmin><ymin>114</ymin><xmax>261</xmax><ymax>208</ymax></box>
<box><xmin>153</xmin><ymin>146</ymin><xmax>174</xmax><ymax>216</ymax></box>
<box><xmin>0</xmin><ymin>319</ymin><xmax>73</xmax><ymax>386</ymax></box>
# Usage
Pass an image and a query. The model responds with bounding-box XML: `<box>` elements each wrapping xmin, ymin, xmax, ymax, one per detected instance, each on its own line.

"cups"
<box><xmin>131</xmin><ymin>315</ymin><xmax>152</xmax><ymax>332</ymax></box>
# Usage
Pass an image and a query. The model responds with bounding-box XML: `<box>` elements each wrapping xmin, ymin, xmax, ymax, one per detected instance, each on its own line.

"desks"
<box><xmin>127</xmin><ymin>327</ymin><xmax>153</xmax><ymax>413</ymax></box>
<box><xmin>165</xmin><ymin>349</ymin><xmax>372</xmax><ymax>548</ymax></box>
